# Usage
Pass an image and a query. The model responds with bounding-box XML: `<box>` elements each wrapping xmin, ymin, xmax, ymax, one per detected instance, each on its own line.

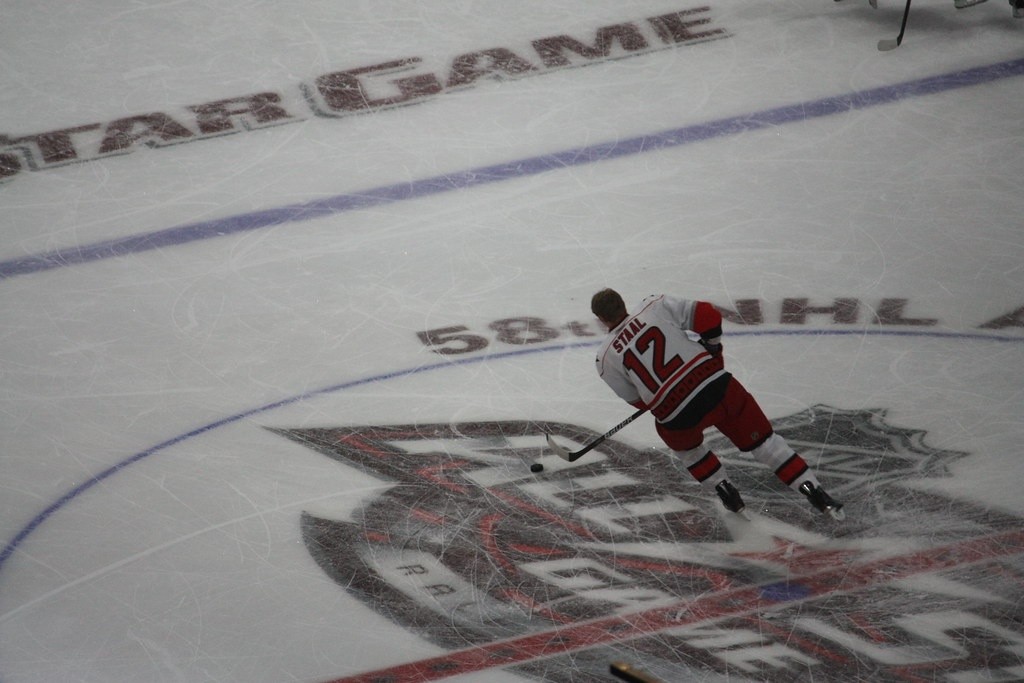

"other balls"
<box><xmin>531</xmin><ymin>464</ymin><xmax>543</xmax><ymax>472</ymax></box>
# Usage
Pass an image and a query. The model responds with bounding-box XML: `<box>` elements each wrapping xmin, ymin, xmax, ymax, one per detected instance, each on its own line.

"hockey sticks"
<box><xmin>877</xmin><ymin>0</ymin><xmax>912</xmax><ymax>51</ymax></box>
<box><xmin>545</xmin><ymin>409</ymin><xmax>646</xmax><ymax>462</ymax></box>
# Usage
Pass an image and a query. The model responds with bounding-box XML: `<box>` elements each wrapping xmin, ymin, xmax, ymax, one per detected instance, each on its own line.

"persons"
<box><xmin>590</xmin><ymin>288</ymin><xmax>846</xmax><ymax>520</ymax></box>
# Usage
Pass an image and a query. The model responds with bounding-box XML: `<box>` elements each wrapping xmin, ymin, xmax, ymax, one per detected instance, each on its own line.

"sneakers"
<box><xmin>714</xmin><ymin>478</ymin><xmax>752</xmax><ymax>522</ymax></box>
<box><xmin>795</xmin><ymin>479</ymin><xmax>847</xmax><ymax>523</ymax></box>
